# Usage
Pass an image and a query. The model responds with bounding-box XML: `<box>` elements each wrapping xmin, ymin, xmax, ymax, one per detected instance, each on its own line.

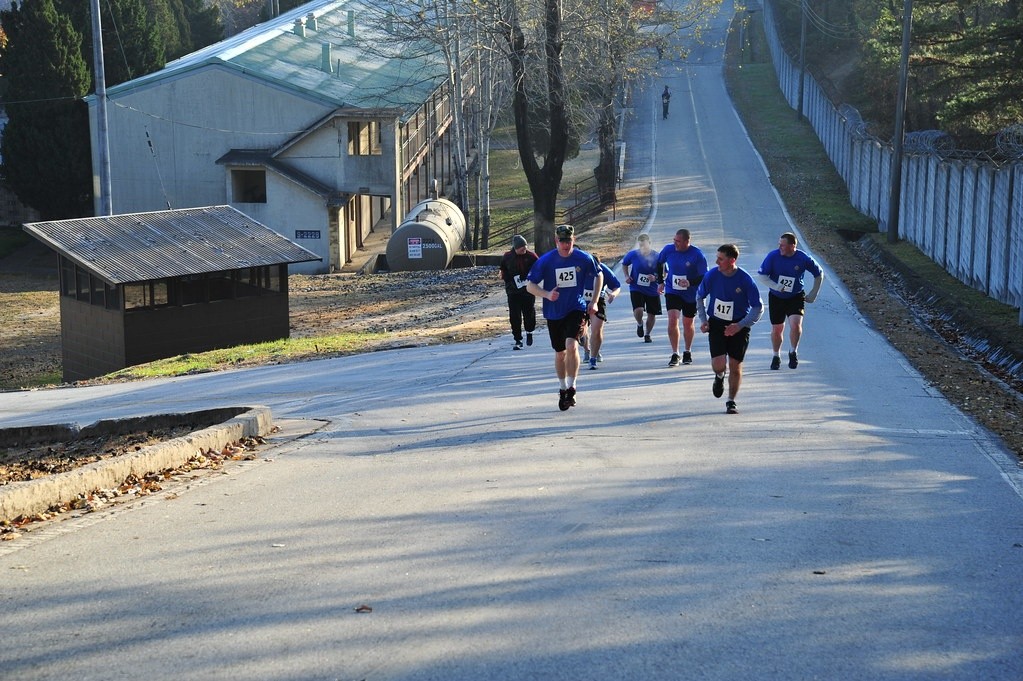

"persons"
<box><xmin>657</xmin><ymin>43</ymin><xmax>663</xmax><ymax>60</ymax></box>
<box><xmin>661</xmin><ymin>85</ymin><xmax>670</xmax><ymax>119</ymax></box>
<box><xmin>759</xmin><ymin>232</ymin><xmax>824</xmax><ymax>370</ymax></box>
<box><xmin>696</xmin><ymin>244</ymin><xmax>764</xmax><ymax>414</ymax></box>
<box><xmin>497</xmin><ymin>225</ymin><xmax>621</xmax><ymax>411</ymax></box>
<box><xmin>621</xmin><ymin>235</ymin><xmax>669</xmax><ymax>343</ymax></box>
<box><xmin>655</xmin><ymin>229</ymin><xmax>709</xmax><ymax>365</ymax></box>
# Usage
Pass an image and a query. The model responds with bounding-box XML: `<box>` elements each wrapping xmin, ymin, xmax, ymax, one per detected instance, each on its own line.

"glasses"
<box><xmin>558</xmin><ymin>238</ymin><xmax>572</xmax><ymax>244</ymax></box>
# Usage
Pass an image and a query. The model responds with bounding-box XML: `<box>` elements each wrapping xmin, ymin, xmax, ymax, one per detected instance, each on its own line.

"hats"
<box><xmin>513</xmin><ymin>235</ymin><xmax>527</xmax><ymax>250</ymax></box>
<box><xmin>555</xmin><ymin>225</ymin><xmax>574</xmax><ymax>238</ymax></box>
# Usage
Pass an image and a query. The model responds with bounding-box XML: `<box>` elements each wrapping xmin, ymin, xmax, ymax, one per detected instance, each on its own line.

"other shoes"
<box><xmin>596</xmin><ymin>353</ymin><xmax>603</xmax><ymax>363</ymax></box>
<box><xmin>526</xmin><ymin>334</ymin><xmax>533</xmax><ymax>346</ymax></box>
<box><xmin>513</xmin><ymin>340</ymin><xmax>523</xmax><ymax>350</ymax></box>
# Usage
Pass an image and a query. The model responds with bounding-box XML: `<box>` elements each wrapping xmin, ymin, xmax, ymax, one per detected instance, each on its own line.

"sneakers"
<box><xmin>788</xmin><ymin>350</ymin><xmax>798</xmax><ymax>369</ymax></box>
<box><xmin>583</xmin><ymin>350</ymin><xmax>590</xmax><ymax>363</ymax></box>
<box><xmin>770</xmin><ymin>356</ymin><xmax>781</xmax><ymax>370</ymax></box>
<box><xmin>588</xmin><ymin>357</ymin><xmax>599</xmax><ymax>370</ymax></box>
<box><xmin>637</xmin><ymin>324</ymin><xmax>644</xmax><ymax>337</ymax></box>
<box><xmin>726</xmin><ymin>401</ymin><xmax>738</xmax><ymax>414</ymax></box>
<box><xmin>682</xmin><ymin>351</ymin><xmax>692</xmax><ymax>364</ymax></box>
<box><xmin>712</xmin><ymin>370</ymin><xmax>725</xmax><ymax>398</ymax></box>
<box><xmin>645</xmin><ymin>335</ymin><xmax>652</xmax><ymax>343</ymax></box>
<box><xmin>559</xmin><ymin>387</ymin><xmax>576</xmax><ymax>411</ymax></box>
<box><xmin>668</xmin><ymin>353</ymin><xmax>682</xmax><ymax>366</ymax></box>
